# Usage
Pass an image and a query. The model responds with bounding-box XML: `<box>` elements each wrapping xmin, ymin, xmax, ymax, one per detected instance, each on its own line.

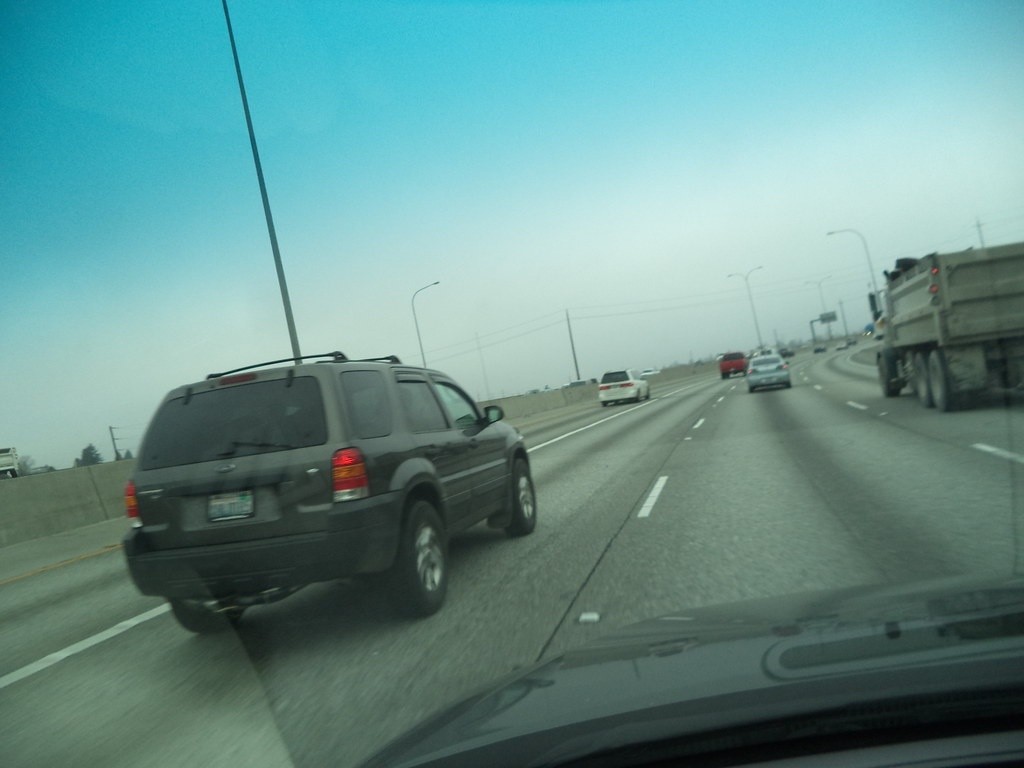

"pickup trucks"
<box><xmin>720</xmin><ymin>353</ymin><xmax>748</xmax><ymax>379</ymax></box>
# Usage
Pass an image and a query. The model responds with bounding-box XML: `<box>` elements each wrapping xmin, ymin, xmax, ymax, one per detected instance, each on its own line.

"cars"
<box><xmin>600</xmin><ymin>371</ymin><xmax>652</xmax><ymax>407</ymax></box>
<box><xmin>835</xmin><ymin>341</ymin><xmax>848</xmax><ymax>350</ymax></box>
<box><xmin>814</xmin><ymin>343</ymin><xmax>827</xmax><ymax>353</ymax></box>
<box><xmin>846</xmin><ymin>338</ymin><xmax>856</xmax><ymax>345</ymax></box>
<box><xmin>748</xmin><ymin>355</ymin><xmax>792</xmax><ymax>394</ymax></box>
<box><xmin>779</xmin><ymin>347</ymin><xmax>795</xmax><ymax>357</ymax></box>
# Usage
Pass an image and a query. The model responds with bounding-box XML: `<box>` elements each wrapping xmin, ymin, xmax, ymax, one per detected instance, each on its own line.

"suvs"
<box><xmin>119</xmin><ymin>349</ymin><xmax>537</xmax><ymax>635</ymax></box>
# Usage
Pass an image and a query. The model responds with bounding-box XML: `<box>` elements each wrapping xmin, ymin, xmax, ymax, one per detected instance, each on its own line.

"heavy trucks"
<box><xmin>870</xmin><ymin>242</ymin><xmax>1024</xmax><ymax>414</ymax></box>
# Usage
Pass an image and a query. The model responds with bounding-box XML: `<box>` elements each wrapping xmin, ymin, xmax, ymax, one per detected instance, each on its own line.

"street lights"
<box><xmin>412</xmin><ymin>280</ymin><xmax>439</xmax><ymax>367</ymax></box>
<box><xmin>803</xmin><ymin>274</ymin><xmax>834</xmax><ymax>339</ymax></box>
<box><xmin>828</xmin><ymin>228</ymin><xmax>878</xmax><ymax>291</ymax></box>
<box><xmin>727</xmin><ymin>266</ymin><xmax>768</xmax><ymax>354</ymax></box>
